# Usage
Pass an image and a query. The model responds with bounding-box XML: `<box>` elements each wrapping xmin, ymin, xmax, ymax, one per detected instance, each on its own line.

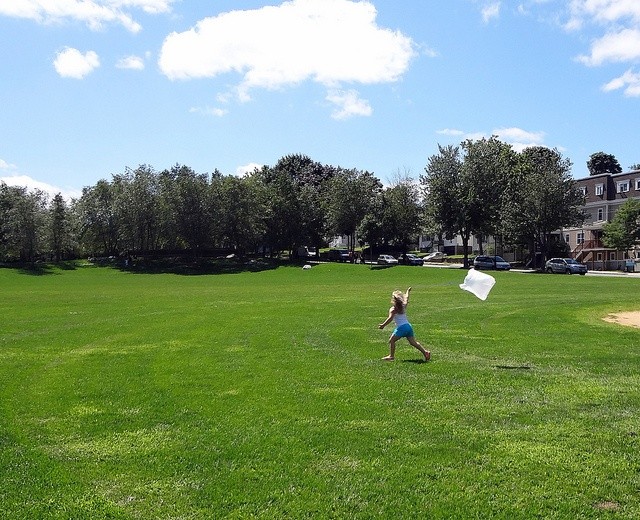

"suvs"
<box><xmin>473</xmin><ymin>256</ymin><xmax>511</xmax><ymax>270</ymax></box>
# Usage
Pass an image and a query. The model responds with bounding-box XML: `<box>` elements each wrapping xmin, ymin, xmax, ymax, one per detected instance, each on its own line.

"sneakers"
<box><xmin>381</xmin><ymin>355</ymin><xmax>395</xmax><ymax>360</ymax></box>
<box><xmin>424</xmin><ymin>351</ymin><xmax>432</xmax><ymax>362</ymax></box>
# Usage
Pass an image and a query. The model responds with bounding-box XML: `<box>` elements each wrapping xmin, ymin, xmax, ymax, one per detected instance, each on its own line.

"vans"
<box><xmin>328</xmin><ymin>249</ymin><xmax>351</xmax><ymax>262</ymax></box>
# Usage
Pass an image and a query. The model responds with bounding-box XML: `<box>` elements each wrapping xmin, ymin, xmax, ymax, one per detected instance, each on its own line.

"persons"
<box><xmin>359</xmin><ymin>252</ymin><xmax>365</xmax><ymax>264</ymax></box>
<box><xmin>354</xmin><ymin>252</ymin><xmax>358</xmax><ymax>264</ymax></box>
<box><xmin>378</xmin><ymin>287</ymin><xmax>430</xmax><ymax>361</ymax></box>
<box><xmin>348</xmin><ymin>250</ymin><xmax>353</xmax><ymax>263</ymax></box>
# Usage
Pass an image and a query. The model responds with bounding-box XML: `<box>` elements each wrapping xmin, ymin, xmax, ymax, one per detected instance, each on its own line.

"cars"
<box><xmin>378</xmin><ymin>254</ymin><xmax>398</xmax><ymax>266</ymax></box>
<box><xmin>544</xmin><ymin>258</ymin><xmax>587</xmax><ymax>276</ymax></box>
<box><xmin>398</xmin><ymin>254</ymin><xmax>424</xmax><ymax>266</ymax></box>
<box><xmin>423</xmin><ymin>252</ymin><xmax>447</xmax><ymax>263</ymax></box>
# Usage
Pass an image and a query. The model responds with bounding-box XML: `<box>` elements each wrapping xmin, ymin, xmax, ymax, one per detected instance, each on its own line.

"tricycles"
<box><xmin>626</xmin><ymin>260</ymin><xmax>634</xmax><ymax>272</ymax></box>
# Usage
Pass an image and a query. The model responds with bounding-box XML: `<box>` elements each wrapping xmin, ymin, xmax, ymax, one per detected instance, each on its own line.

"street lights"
<box><xmin>582</xmin><ymin>193</ymin><xmax>589</xmax><ymax>266</ymax></box>
